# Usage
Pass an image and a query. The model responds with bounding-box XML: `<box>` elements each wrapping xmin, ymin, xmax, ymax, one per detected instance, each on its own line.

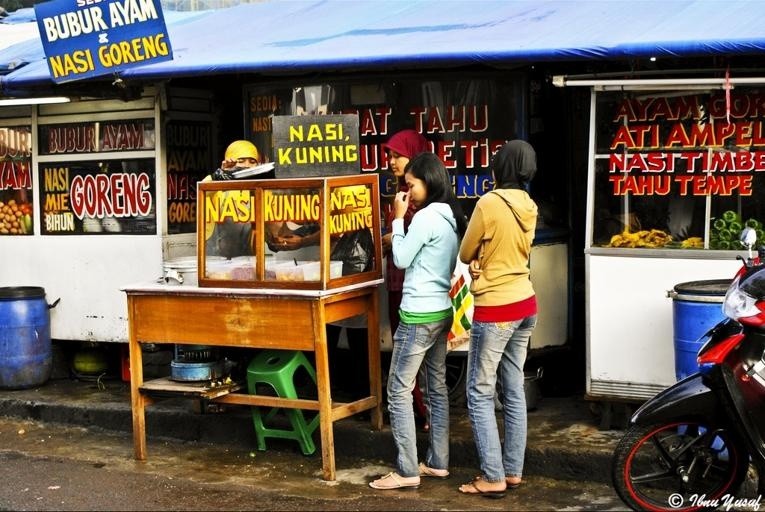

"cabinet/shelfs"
<box><xmin>195</xmin><ymin>170</ymin><xmax>383</xmax><ymax>290</ymax></box>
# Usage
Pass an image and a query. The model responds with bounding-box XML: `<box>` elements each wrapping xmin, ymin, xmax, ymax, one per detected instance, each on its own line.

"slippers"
<box><xmin>368</xmin><ymin>463</ymin><xmax>522</xmax><ymax>499</ymax></box>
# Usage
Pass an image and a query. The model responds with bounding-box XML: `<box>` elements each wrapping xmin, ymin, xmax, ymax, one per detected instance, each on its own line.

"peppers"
<box><xmin>710</xmin><ymin>210</ymin><xmax>763</xmax><ymax>249</ymax></box>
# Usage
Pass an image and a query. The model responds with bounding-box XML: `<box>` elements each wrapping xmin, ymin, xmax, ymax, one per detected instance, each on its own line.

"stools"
<box><xmin>246</xmin><ymin>346</ymin><xmax>331</xmax><ymax>457</ymax></box>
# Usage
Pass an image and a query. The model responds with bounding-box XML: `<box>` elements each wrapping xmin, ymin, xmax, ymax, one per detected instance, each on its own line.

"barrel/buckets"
<box><xmin>2</xmin><ymin>285</ymin><xmax>59</xmax><ymax>391</ymax></box>
<box><xmin>667</xmin><ymin>279</ymin><xmax>745</xmax><ymax>462</ymax></box>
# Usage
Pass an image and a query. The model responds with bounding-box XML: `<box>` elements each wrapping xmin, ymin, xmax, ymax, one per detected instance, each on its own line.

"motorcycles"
<box><xmin>608</xmin><ymin>223</ymin><xmax>764</xmax><ymax>510</ymax></box>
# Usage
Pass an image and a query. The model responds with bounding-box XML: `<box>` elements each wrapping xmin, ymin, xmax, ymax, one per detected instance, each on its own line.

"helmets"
<box><xmin>224</xmin><ymin>140</ymin><xmax>259</xmax><ymax>165</ymax></box>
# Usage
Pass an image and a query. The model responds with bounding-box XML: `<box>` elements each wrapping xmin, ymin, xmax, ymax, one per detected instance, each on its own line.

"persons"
<box><xmin>202</xmin><ymin>141</ymin><xmax>256</xmax><ymax>257</ymax></box>
<box><xmin>458</xmin><ymin>140</ymin><xmax>538</xmax><ymax>500</ymax></box>
<box><xmin>367</xmin><ymin>152</ymin><xmax>469</xmax><ymax>492</ymax></box>
<box><xmin>636</xmin><ymin>146</ymin><xmax>725</xmax><ymax>239</ymax></box>
<box><xmin>382</xmin><ymin>128</ymin><xmax>433</xmax><ymax>433</ymax></box>
<box><xmin>263</xmin><ymin>186</ymin><xmax>373</xmax><ymax>422</ymax></box>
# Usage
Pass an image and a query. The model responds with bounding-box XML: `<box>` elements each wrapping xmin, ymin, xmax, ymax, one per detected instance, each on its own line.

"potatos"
<box><xmin>0</xmin><ymin>199</ymin><xmax>32</xmax><ymax>235</ymax></box>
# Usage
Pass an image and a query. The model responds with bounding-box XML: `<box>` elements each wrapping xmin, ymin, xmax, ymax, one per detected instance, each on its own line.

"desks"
<box><xmin>120</xmin><ymin>274</ymin><xmax>386</xmax><ymax>489</ymax></box>
